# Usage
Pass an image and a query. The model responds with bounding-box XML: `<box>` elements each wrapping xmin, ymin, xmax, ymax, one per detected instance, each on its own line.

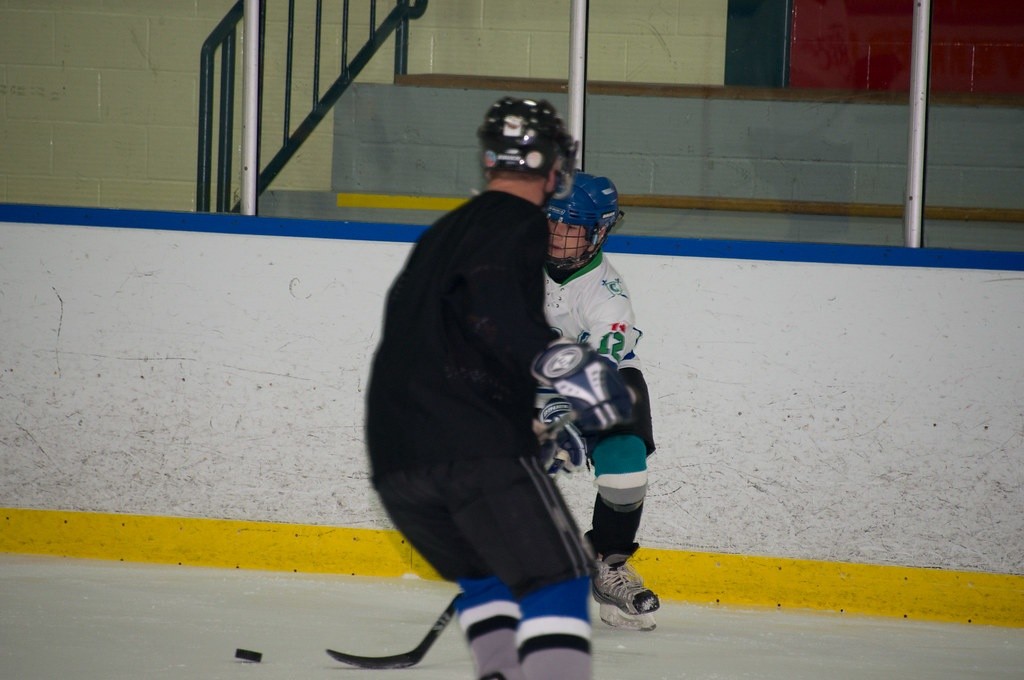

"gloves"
<box><xmin>538</xmin><ymin>403</ymin><xmax>586</xmax><ymax>475</ymax></box>
<box><xmin>531</xmin><ymin>334</ymin><xmax>635</xmax><ymax>429</ymax></box>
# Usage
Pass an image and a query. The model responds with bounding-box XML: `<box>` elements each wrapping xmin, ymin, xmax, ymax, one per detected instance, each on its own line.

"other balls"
<box><xmin>235</xmin><ymin>649</ymin><xmax>262</xmax><ymax>663</ymax></box>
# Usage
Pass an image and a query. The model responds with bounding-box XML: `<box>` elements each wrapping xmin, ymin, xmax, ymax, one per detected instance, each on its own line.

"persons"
<box><xmin>545</xmin><ymin>169</ymin><xmax>660</xmax><ymax>629</ymax></box>
<box><xmin>366</xmin><ymin>97</ymin><xmax>632</xmax><ymax>680</ymax></box>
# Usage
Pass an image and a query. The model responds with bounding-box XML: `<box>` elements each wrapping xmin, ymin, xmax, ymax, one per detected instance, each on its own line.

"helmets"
<box><xmin>543</xmin><ymin>169</ymin><xmax>620</xmax><ymax>284</ymax></box>
<box><xmin>476</xmin><ymin>95</ymin><xmax>578</xmax><ymax>176</ymax></box>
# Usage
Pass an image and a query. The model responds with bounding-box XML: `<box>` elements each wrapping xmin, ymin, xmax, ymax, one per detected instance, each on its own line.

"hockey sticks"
<box><xmin>537</xmin><ymin>411</ymin><xmax>578</xmax><ymax>443</ymax></box>
<box><xmin>326</xmin><ymin>450</ymin><xmax>570</xmax><ymax>668</ymax></box>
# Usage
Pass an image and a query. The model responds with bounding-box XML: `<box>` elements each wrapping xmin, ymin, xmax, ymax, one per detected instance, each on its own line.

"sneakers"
<box><xmin>584</xmin><ymin>528</ymin><xmax>661</xmax><ymax>631</ymax></box>
